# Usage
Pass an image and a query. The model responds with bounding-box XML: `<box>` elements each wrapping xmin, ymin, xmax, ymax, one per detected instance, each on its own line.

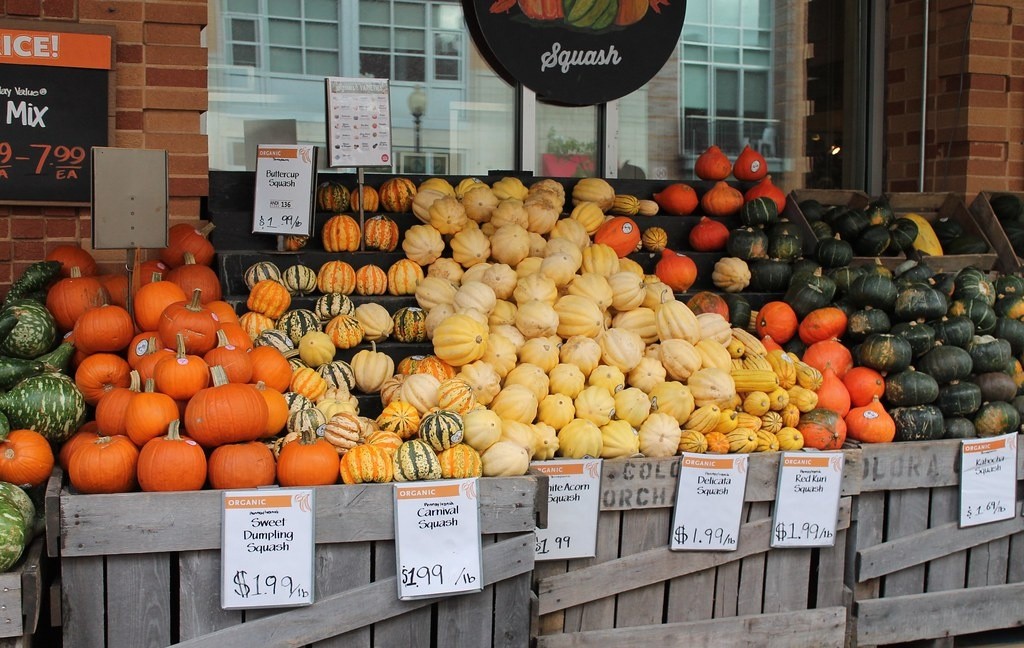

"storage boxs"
<box><xmin>1</xmin><ymin>169</ymin><xmax>1024</xmax><ymax>648</ymax></box>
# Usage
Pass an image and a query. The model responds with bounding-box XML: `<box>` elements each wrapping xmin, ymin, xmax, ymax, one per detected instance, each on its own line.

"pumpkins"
<box><xmin>0</xmin><ymin>144</ymin><xmax>1023</xmax><ymax>573</ymax></box>
